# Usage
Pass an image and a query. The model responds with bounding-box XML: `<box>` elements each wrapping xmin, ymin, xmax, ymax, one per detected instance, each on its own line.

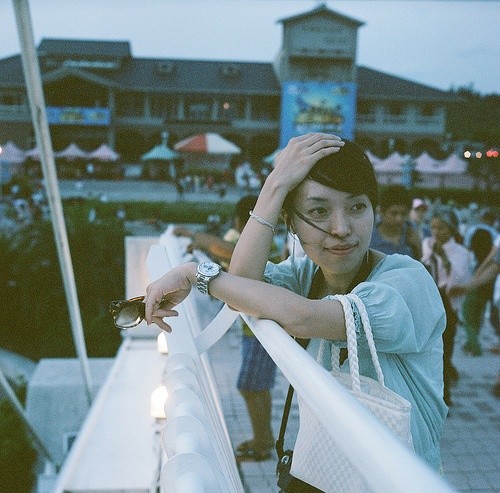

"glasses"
<box><xmin>108</xmin><ymin>296</ymin><xmax>164</xmax><ymax>329</ymax></box>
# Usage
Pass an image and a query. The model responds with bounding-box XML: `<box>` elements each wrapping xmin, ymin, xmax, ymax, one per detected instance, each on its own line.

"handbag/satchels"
<box><xmin>276</xmin><ymin>450</ymin><xmax>323</xmax><ymax>493</ymax></box>
<box><xmin>289</xmin><ymin>293</ymin><xmax>415</xmax><ymax>493</ymax></box>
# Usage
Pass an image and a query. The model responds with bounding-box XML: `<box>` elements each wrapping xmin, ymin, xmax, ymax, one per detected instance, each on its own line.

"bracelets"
<box><xmin>249</xmin><ymin>211</ymin><xmax>276</xmax><ymax>232</ymax></box>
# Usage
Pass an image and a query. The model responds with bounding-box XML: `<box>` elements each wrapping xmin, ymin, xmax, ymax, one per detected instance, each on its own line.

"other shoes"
<box><xmin>235</xmin><ymin>440</ymin><xmax>275</xmax><ymax>461</ymax></box>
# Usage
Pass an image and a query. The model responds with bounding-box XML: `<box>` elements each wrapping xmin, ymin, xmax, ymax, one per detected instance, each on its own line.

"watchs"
<box><xmin>194</xmin><ymin>261</ymin><xmax>221</xmax><ymax>296</ymax></box>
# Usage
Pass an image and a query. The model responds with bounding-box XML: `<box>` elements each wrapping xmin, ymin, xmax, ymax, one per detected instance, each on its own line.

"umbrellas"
<box><xmin>366</xmin><ymin>150</ymin><xmax>468</xmax><ymax>186</ymax></box>
<box><xmin>0</xmin><ymin>134</ymin><xmax>242</xmax><ymax>177</ymax></box>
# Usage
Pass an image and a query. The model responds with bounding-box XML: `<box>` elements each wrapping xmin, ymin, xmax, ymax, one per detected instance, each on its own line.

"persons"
<box><xmin>0</xmin><ymin>156</ymin><xmax>500</xmax><ymax>463</ymax></box>
<box><xmin>142</xmin><ymin>133</ymin><xmax>450</xmax><ymax>493</ymax></box>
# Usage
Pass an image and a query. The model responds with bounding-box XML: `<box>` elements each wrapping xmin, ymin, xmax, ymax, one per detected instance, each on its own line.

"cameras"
<box><xmin>277</xmin><ymin>450</ymin><xmax>325</xmax><ymax>493</ymax></box>
